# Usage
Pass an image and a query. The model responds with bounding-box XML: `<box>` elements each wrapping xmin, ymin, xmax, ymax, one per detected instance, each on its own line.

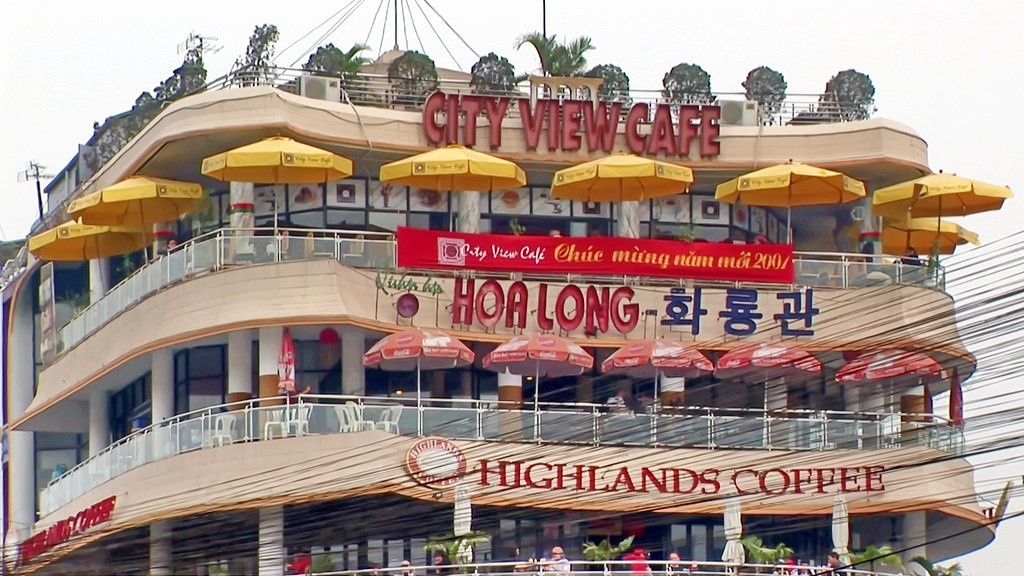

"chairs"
<box><xmin>200</xmin><ymin>401</ymin><xmax>404</xmax><ymax>450</ymax></box>
<box><xmin>219</xmin><ymin>227</ymin><xmax>397</xmax><ymax>274</ymax></box>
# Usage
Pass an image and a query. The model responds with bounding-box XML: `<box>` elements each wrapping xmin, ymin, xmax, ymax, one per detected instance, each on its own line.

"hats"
<box><xmin>400</xmin><ymin>560</ymin><xmax>410</xmax><ymax>565</ymax></box>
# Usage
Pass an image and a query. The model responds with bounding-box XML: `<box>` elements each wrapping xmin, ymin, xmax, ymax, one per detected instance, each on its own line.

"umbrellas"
<box><xmin>602</xmin><ymin>336</ymin><xmax>715</xmax><ymax>447</ymax></box>
<box><xmin>278</xmin><ymin>327</ymin><xmax>296</xmax><ymax>434</ymax></box>
<box><xmin>483</xmin><ymin>331</ymin><xmax>593</xmax><ymax>439</ymax></box>
<box><xmin>832</xmin><ymin>493</ymin><xmax>853</xmax><ymax>576</ymax></box>
<box><xmin>835</xmin><ymin>349</ymin><xmax>949</xmax><ymax>443</ymax></box>
<box><xmin>363</xmin><ymin>327</ymin><xmax>475</xmax><ymax>435</ymax></box>
<box><xmin>722</xmin><ymin>481</ymin><xmax>746</xmax><ymax>572</ymax></box>
<box><xmin>873</xmin><ymin>169</ymin><xmax>1015</xmax><ymax>289</ymax></box>
<box><xmin>378</xmin><ymin>143</ymin><xmax>527</xmax><ymax>232</ymax></box>
<box><xmin>713</xmin><ymin>337</ymin><xmax>822</xmax><ymax>446</ymax></box>
<box><xmin>453</xmin><ymin>478</ymin><xmax>472</xmax><ymax>573</ymax></box>
<box><xmin>550</xmin><ymin>152</ymin><xmax>694</xmax><ymax>235</ymax></box>
<box><xmin>201</xmin><ymin>135</ymin><xmax>353</xmax><ymax>262</ymax></box>
<box><xmin>715</xmin><ymin>159</ymin><xmax>867</xmax><ymax>245</ymax></box>
<box><xmin>28</xmin><ymin>172</ymin><xmax>211</xmax><ymax>265</ymax></box>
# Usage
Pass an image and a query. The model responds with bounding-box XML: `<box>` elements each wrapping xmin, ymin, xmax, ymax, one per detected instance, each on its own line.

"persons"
<box><xmin>160</xmin><ymin>239</ymin><xmax>184</xmax><ymax>256</ymax></box>
<box><xmin>544</xmin><ymin>546</ymin><xmax>572</xmax><ymax>574</ymax></box>
<box><xmin>549</xmin><ymin>229</ymin><xmax>770</xmax><ymax>284</ymax></box>
<box><xmin>285</xmin><ymin>555</ymin><xmax>312</xmax><ymax>574</ymax></box>
<box><xmin>610</xmin><ymin>387</ymin><xmax>654</xmax><ymax>415</ymax></box>
<box><xmin>624</xmin><ymin>547</ymin><xmax>688</xmax><ymax>576</ymax></box>
<box><xmin>780</xmin><ymin>552</ymin><xmax>848</xmax><ymax>576</ymax></box>
<box><xmin>365</xmin><ymin>550</ymin><xmax>453</xmax><ymax>576</ymax></box>
<box><xmin>857</xmin><ymin>242</ymin><xmax>921</xmax><ymax>286</ymax></box>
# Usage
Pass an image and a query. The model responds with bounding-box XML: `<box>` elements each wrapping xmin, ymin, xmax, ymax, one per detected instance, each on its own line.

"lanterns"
<box><xmin>319</xmin><ymin>328</ymin><xmax>340</xmax><ymax>347</ymax></box>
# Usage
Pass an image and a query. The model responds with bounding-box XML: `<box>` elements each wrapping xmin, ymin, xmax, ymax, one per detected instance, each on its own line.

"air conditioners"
<box><xmin>714</xmin><ymin>100</ymin><xmax>759</xmax><ymax>126</ymax></box>
<box><xmin>296</xmin><ymin>76</ymin><xmax>340</xmax><ymax>102</ymax></box>
